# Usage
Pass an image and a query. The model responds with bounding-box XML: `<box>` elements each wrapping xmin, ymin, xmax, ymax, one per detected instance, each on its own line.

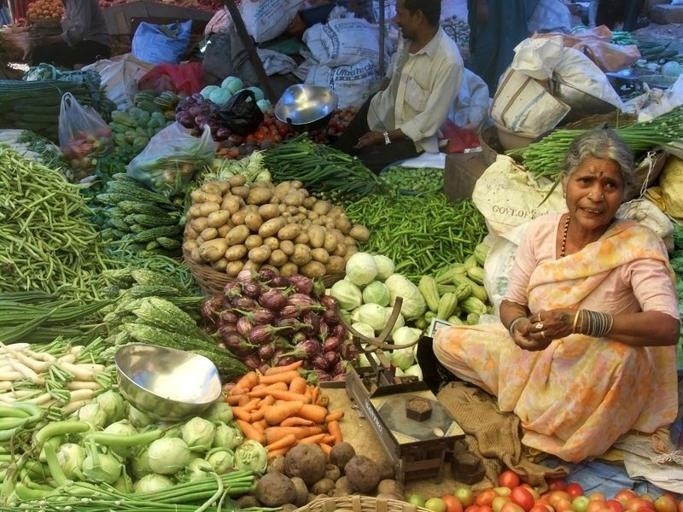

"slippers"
<box><xmin>651</xmin><ymin>427</ymin><xmax>678</xmax><ymax>455</ymax></box>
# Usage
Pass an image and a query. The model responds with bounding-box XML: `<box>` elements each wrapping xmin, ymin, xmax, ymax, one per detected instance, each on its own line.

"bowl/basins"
<box><xmin>553</xmin><ymin>76</ymin><xmax>617</xmax><ymax>116</ymax></box>
<box><xmin>494</xmin><ymin>126</ymin><xmax>538</xmax><ymax>165</ymax></box>
<box><xmin>113</xmin><ymin>344</ymin><xmax>223</xmax><ymax>422</ymax></box>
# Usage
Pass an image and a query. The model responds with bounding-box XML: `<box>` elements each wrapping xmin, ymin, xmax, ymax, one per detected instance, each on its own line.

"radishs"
<box><xmin>0</xmin><ymin>340</ymin><xmax>106</xmax><ymax>416</ymax></box>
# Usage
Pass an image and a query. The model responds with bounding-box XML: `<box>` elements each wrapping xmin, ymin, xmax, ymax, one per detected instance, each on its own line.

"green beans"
<box><xmin>0</xmin><ymin>140</ymin><xmax>115</xmax><ymax>307</ymax></box>
<box><xmin>260</xmin><ymin>130</ymin><xmax>489</xmax><ymax>278</ymax></box>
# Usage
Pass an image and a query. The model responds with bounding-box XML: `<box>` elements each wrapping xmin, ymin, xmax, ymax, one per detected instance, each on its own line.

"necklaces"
<box><xmin>560</xmin><ymin>215</ymin><xmax>570</xmax><ymax>258</ymax></box>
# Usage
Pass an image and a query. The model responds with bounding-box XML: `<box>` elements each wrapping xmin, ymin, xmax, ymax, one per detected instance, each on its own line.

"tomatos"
<box><xmin>247</xmin><ymin>104</ymin><xmax>356</xmax><ymax>150</ymax></box>
<box><xmin>409</xmin><ymin>470</ymin><xmax>683</xmax><ymax>512</ymax></box>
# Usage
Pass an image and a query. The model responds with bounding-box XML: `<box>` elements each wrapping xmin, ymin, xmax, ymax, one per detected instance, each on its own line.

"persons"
<box><xmin>334</xmin><ymin>0</ymin><xmax>466</xmax><ymax>178</ymax></box>
<box><xmin>430</xmin><ymin>128</ymin><xmax>682</xmax><ymax>466</ymax></box>
<box><xmin>35</xmin><ymin>0</ymin><xmax>113</xmax><ymax>68</ymax></box>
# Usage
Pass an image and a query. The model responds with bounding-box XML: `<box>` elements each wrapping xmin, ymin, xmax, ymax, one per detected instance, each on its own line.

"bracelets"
<box><xmin>571</xmin><ymin>309</ymin><xmax>580</xmax><ymax>334</ymax></box>
<box><xmin>579</xmin><ymin>309</ymin><xmax>614</xmax><ymax>337</ymax></box>
<box><xmin>509</xmin><ymin>316</ymin><xmax>529</xmax><ymax>336</ymax></box>
<box><xmin>382</xmin><ymin>131</ymin><xmax>391</xmax><ymax>146</ymax></box>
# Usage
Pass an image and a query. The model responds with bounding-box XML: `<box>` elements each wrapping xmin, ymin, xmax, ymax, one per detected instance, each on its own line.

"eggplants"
<box><xmin>202</xmin><ymin>265</ymin><xmax>359</xmax><ymax>380</ymax></box>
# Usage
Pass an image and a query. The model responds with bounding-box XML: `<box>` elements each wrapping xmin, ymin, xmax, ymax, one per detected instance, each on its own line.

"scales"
<box><xmin>330</xmin><ymin>295</ymin><xmax>468</xmax><ymax>485</ymax></box>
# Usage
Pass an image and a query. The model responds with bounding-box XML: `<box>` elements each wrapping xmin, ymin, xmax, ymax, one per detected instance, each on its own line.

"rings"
<box><xmin>541</xmin><ymin>331</ymin><xmax>545</xmax><ymax>339</ymax></box>
<box><xmin>536</xmin><ymin>322</ymin><xmax>543</xmax><ymax>331</ymax></box>
<box><xmin>538</xmin><ymin>312</ymin><xmax>542</xmax><ymax>321</ymax></box>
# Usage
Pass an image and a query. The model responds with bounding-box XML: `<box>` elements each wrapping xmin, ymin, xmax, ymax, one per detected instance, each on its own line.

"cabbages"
<box><xmin>328</xmin><ymin>251</ymin><xmax>425</xmax><ymax>380</ymax></box>
<box><xmin>55</xmin><ymin>387</ymin><xmax>268</xmax><ymax>494</ymax></box>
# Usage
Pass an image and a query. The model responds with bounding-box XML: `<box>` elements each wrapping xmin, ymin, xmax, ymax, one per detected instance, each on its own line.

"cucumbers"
<box><xmin>418</xmin><ymin>240</ymin><xmax>488</xmax><ymax>327</ymax></box>
<box><xmin>0</xmin><ymin>402</ymin><xmax>178</xmax><ymax>500</ymax></box>
<box><xmin>0</xmin><ymin>290</ymin><xmax>126</xmax><ymax>343</ymax></box>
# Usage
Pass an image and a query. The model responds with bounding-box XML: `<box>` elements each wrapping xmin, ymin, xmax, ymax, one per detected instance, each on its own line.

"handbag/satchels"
<box><xmin>131</xmin><ymin>20</ymin><xmax>193</xmax><ymax>64</ymax></box>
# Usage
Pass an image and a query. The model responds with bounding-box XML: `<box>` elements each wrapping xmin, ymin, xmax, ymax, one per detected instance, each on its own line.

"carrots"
<box><xmin>224</xmin><ymin>359</ymin><xmax>344</xmax><ymax>458</ymax></box>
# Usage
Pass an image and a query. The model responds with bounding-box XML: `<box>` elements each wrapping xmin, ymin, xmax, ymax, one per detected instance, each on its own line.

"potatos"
<box><xmin>239</xmin><ymin>443</ymin><xmax>406</xmax><ymax>512</ymax></box>
<box><xmin>182</xmin><ymin>174</ymin><xmax>369</xmax><ymax>277</ymax></box>
